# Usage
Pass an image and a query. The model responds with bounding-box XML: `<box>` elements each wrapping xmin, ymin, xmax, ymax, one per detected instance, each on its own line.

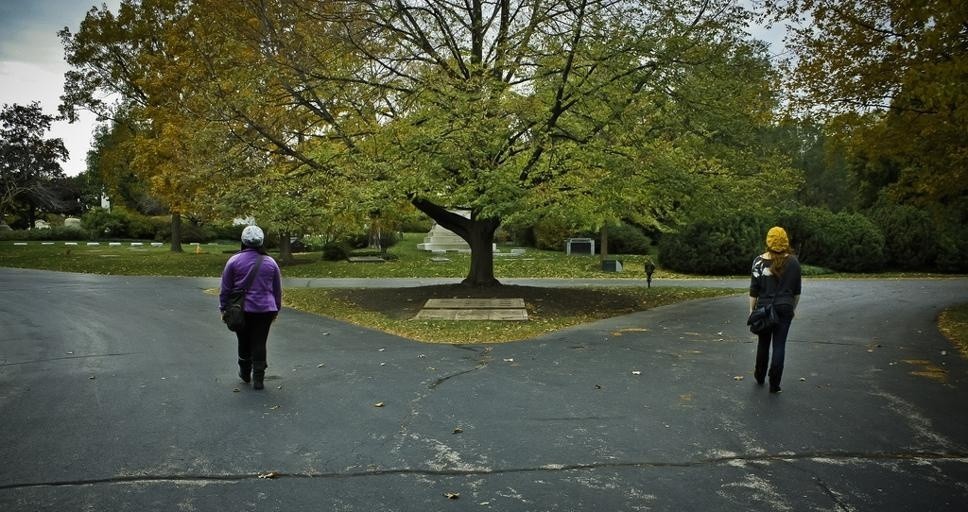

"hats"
<box><xmin>240</xmin><ymin>226</ymin><xmax>266</xmax><ymax>250</ymax></box>
<box><xmin>766</xmin><ymin>226</ymin><xmax>789</xmax><ymax>254</ymax></box>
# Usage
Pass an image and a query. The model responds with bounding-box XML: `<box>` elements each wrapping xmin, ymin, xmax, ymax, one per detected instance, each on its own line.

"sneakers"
<box><xmin>753</xmin><ymin>372</ymin><xmax>765</xmax><ymax>385</ymax></box>
<box><xmin>770</xmin><ymin>387</ymin><xmax>783</xmax><ymax>393</ymax></box>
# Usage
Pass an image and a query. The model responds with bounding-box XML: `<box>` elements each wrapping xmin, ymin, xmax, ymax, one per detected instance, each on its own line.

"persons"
<box><xmin>217</xmin><ymin>225</ymin><xmax>282</xmax><ymax>391</ymax></box>
<box><xmin>749</xmin><ymin>225</ymin><xmax>803</xmax><ymax>394</ymax></box>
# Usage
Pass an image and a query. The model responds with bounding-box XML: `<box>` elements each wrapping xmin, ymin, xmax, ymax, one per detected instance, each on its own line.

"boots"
<box><xmin>237</xmin><ymin>356</ymin><xmax>252</xmax><ymax>383</ymax></box>
<box><xmin>252</xmin><ymin>360</ymin><xmax>268</xmax><ymax>391</ymax></box>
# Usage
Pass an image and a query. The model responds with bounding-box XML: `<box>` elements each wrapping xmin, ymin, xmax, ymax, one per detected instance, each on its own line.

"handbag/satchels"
<box><xmin>746</xmin><ymin>301</ymin><xmax>777</xmax><ymax>337</ymax></box>
<box><xmin>224</xmin><ymin>256</ymin><xmax>262</xmax><ymax>332</ymax></box>
